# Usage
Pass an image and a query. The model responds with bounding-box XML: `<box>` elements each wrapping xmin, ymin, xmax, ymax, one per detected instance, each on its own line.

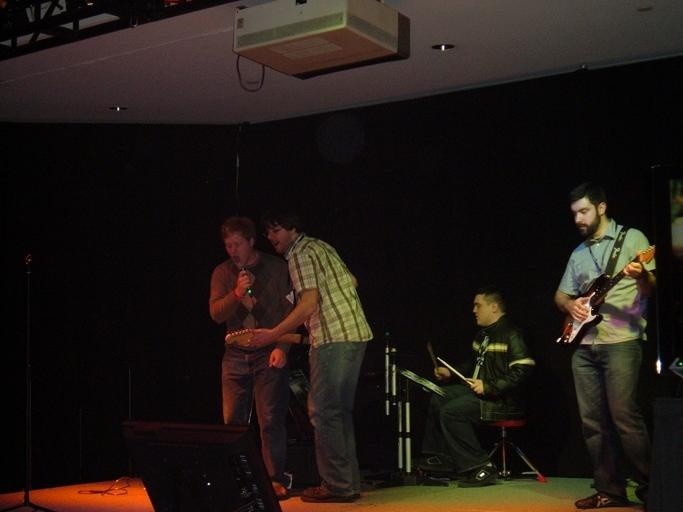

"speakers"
<box><xmin>649</xmin><ymin>396</ymin><xmax>683</xmax><ymax>512</ymax></box>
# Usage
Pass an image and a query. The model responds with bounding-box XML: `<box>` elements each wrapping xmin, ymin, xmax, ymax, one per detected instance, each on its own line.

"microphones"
<box><xmin>239</xmin><ymin>265</ymin><xmax>254</xmax><ymax>297</ymax></box>
<box><xmin>25</xmin><ymin>249</ymin><xmax>33</xmax><ymax>268</ymax></box>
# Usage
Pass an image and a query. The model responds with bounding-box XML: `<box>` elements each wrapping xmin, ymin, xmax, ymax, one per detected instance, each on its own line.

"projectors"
<box><xmin>232</xmin><ymin>0</ymin><xmax>410</xmax><ymax>81</ymax></box>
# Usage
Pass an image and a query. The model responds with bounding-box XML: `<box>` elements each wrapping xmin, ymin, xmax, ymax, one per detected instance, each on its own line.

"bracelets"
<box><xmin>232</xmin><ymin>287</ymin><xmax>245</xmax><ymax>301</ymax></box>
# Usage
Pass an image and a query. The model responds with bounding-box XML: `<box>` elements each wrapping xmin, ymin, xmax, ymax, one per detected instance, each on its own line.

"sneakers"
<box><xmin>271</xmin><ymin>481</ymin><xmax>288</xmax><ymax>500</ymax></box>
<box><xmin>574</xmin><ymin>491</ymin><xmax>627</xmax><ymax>509</ymax></box>
<box><xmin>459</xmin><ymin>464</ymin><xmax>498</xmax><ymax>487</ymax></box>
<box><xmin>301</xmin><ymin>488</ymin><xmax>355</xmax><ymax>503</ymax></box>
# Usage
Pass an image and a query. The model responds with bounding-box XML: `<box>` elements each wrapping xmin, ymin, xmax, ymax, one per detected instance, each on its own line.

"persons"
<box><xmin>553</xmin><ymin>182</ymin><xmax>658</xmax><ymax>510</ymax></box>
<box><xmin>414</xmin><ymin>283</ymin><xmax>537</xmax><ymax>490</ymax></box>
<box><xmin>248</xmin><ymin>211</ymin><xmax>374</xmax><ymax>505</ymax></box>
<box><xmin>209</xmin><ymin>215</ymin><xmax>296</xmax><ymax>502</ymax></box>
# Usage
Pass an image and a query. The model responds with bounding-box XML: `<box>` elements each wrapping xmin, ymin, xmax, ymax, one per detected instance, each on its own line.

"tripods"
<box><xmin>375</xmin><ymin>399</ymin><xmax>449</xmax><ymax>489</ymax></box>
<box><xmin>0</xmin><ymin>276</ymin><xmax>55</xmax><ymax>512</ymax></box>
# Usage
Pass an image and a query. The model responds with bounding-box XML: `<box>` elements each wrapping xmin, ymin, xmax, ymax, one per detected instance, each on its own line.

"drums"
<box><xmin>401</xmin><ymin>368</ymin><xmax>445</xmax><ymax>397</ymax></box>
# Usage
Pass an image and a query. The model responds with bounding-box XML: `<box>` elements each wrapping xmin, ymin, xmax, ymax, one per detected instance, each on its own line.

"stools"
<box><xmin>485</xmin><ymin>419</ymin><xmax>545</xmax><ymax>481</ymax></box>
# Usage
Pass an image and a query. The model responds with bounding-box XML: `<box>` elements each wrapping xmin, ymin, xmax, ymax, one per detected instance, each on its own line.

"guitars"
<box><xmin>223</xmin><ymin>327</ymin><xmax>308</xmax><ymax>352</ymax></box>
<box><xmin>556</xmin><ymin>246</ymin><xmax>655</xmax><ymax>351</ymax></box>
